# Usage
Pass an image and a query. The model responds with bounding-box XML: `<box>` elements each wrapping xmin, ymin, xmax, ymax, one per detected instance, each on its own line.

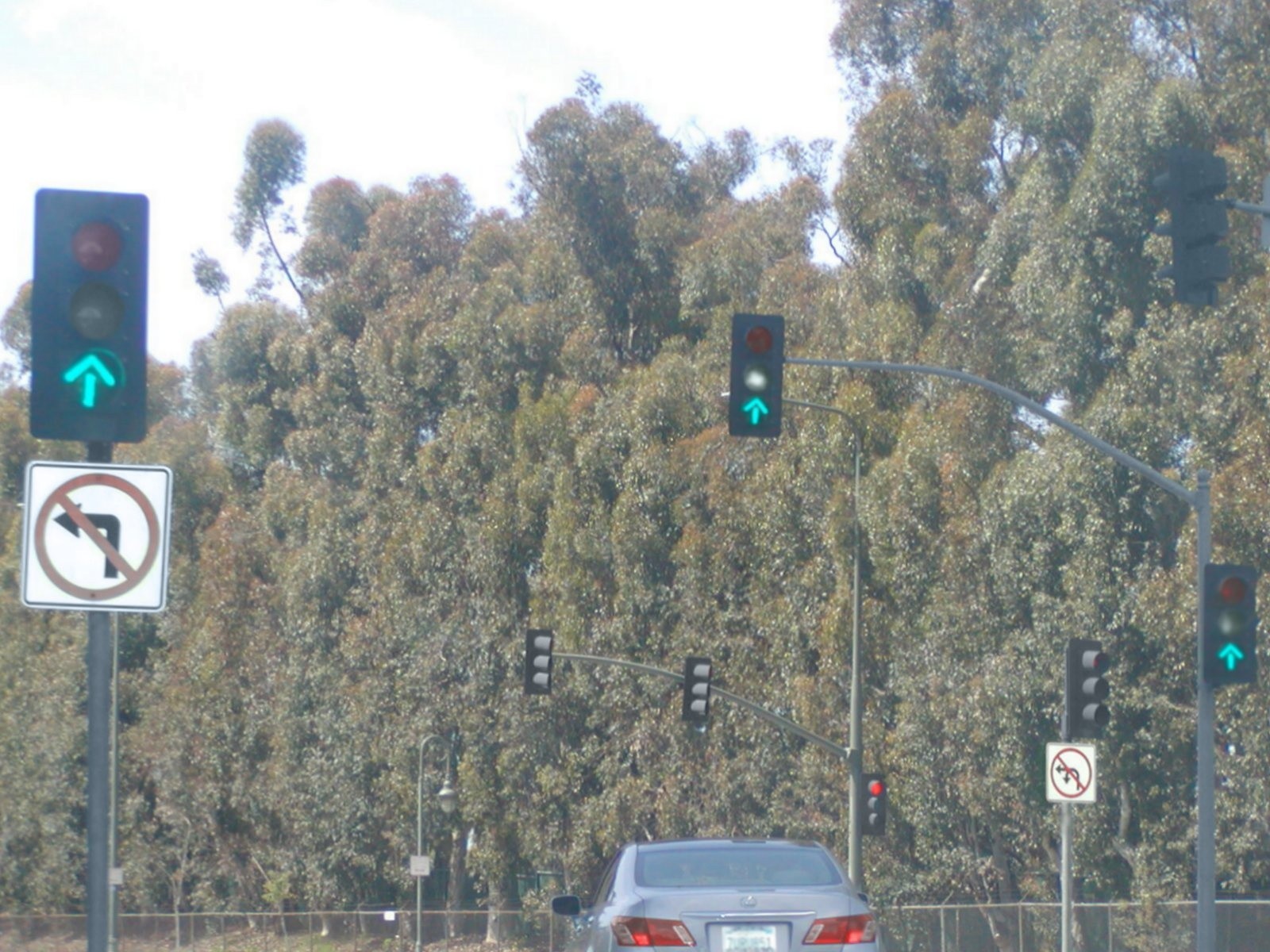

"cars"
<box><xmin>552</xmin><ymin>838</ymin><xmax>883</xmax><ymax>951</ymax></box>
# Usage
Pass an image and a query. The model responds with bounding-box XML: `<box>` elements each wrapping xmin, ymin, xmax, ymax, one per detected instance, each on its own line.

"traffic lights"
<box><xmin>24</xmin><ymin>188</ymin><xmax>148</xmax><ymax>441</ymax></box>
<box><xmin>524</xmin><ymin>628</ymin><xmax>555</xmax><ymax>693</ymax></box>
<box><xmin>728</xmin><ymin>314</ymin><xmax>784</xmax><ymax>436</ymax></box>
<box><xmin>859</xmin><ymin>772</ymin><xmax>892</xmax><ymax>839</ymax></box>
<box><xmin>682</xmin><ymin>656</ymin><xmax>713</xmax><ymax>723</ymax></box>
<box><xmin>1202</xmin><ymin>562</ymin><xmax>1266</xmax><ymax>690</ymax></box>
<box><xmin>1060</xmin><ymin>638</ymin><xmax>1112</xmax><ymax>741</ymax></box>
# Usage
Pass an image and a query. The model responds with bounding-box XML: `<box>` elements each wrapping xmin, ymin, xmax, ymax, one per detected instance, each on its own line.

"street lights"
<box><xmin>721</xmin><ymin>391</ymin><xmax>871</xmax><ymax>897</ymax></box>
<box><xmin>413</xmin><ymin>735</ymin><xmax>461</xmax><ymax>951</ymax></box>
